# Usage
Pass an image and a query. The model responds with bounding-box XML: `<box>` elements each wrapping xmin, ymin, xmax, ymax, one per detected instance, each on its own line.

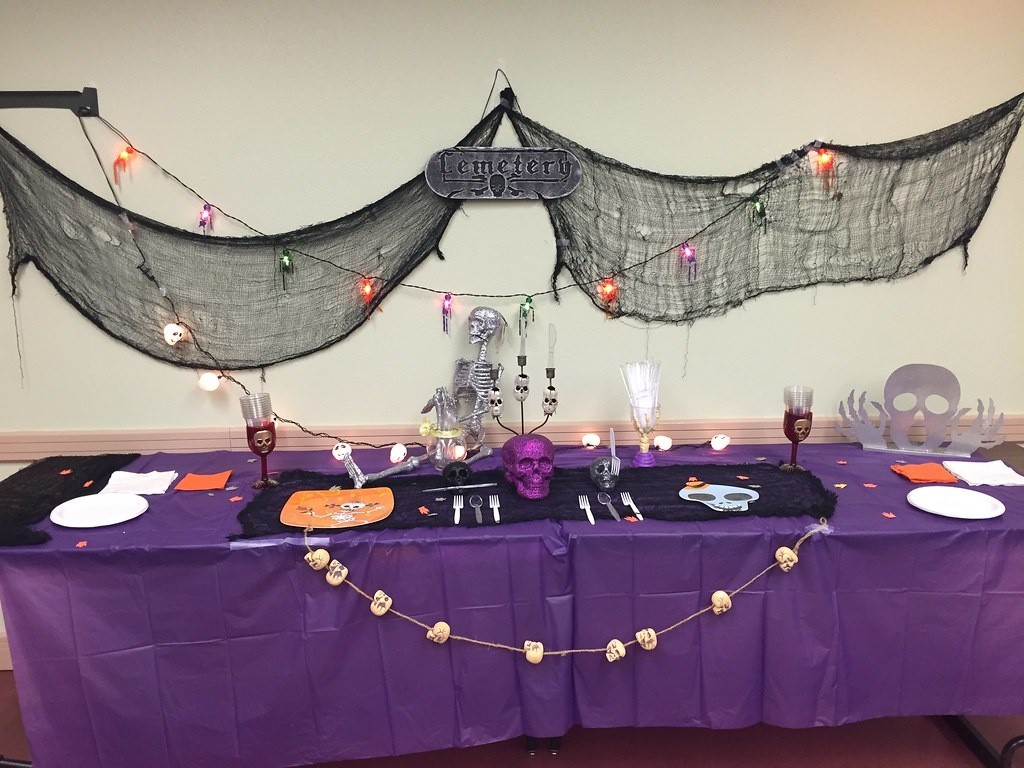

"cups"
<box><xmin>784</xmin><ymin>384</ymin><xmax>814</xmax><ymax>415</ymax></box>
<box><xmin>239</xmin><ymin>392</ymin><xmax>272</xmax><ymax>428</ymax></box>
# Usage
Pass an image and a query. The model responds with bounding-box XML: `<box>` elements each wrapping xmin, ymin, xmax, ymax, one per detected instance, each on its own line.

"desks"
<box><xmin>0</xmin><ymin>444</ymin><xmax>1024</xmax><ymax>768</ymax></box>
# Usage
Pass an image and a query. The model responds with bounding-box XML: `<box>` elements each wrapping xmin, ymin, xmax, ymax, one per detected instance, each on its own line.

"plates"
<box><xmin>49</xmin><ymin>492</ymin><xmax>150</xmax><ymax>528</ymax></box>
<box><xmin>907</xmin><ymin>485</ymin><xmax>1006</xmax><ymax>520</ymax></box>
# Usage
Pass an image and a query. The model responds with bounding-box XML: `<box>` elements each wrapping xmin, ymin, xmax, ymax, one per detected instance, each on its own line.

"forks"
<box><xmin>489</xmin><ymin>494</ymin><xmax>501</xmax><ymax>524</ymax></box>
<box><xmin>609</xmin><ymin>428</ymin><xmax>621</xmax><ymax>476</ymax></box>
<box><xmin>620</xmin><ymin>491</ymin><xmax>644</xmax><ymax>521</ymax></box>
<box><xmin>579</xmin><ymin>494</ymin><xmax>595</xmax><ymax>526</ymax></box>
<box><xmin>453</xmin><ymin>495</ymin><xmax>464</xmax><ymax>524</ymax></box>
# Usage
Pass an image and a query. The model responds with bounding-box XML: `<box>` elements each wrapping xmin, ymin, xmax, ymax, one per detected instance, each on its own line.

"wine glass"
<box><xmin>246</xmin><ymin>422</ymin><xmax>280</xmax><ymax>488</ymax></box>
<box><xmin>630</xmin><ymin>402</ymin><xmax>662</xmax><ymax>469</ymax></box>
<box><xmin>779</xmin><ymin>408</ymin><xmax>813</xmax><ymax>473</ymax></box>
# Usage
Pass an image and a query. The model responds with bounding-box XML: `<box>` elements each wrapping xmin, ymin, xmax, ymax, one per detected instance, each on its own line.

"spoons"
<box><xmin>469</xmin><ymin>494</ymin><xmax>483</xmax><ymax>524</ymax></box>
<box><xmin>597</xmin><ymin>492</ymin><xmax>621</xmax><ymax>522</ymax></box>
